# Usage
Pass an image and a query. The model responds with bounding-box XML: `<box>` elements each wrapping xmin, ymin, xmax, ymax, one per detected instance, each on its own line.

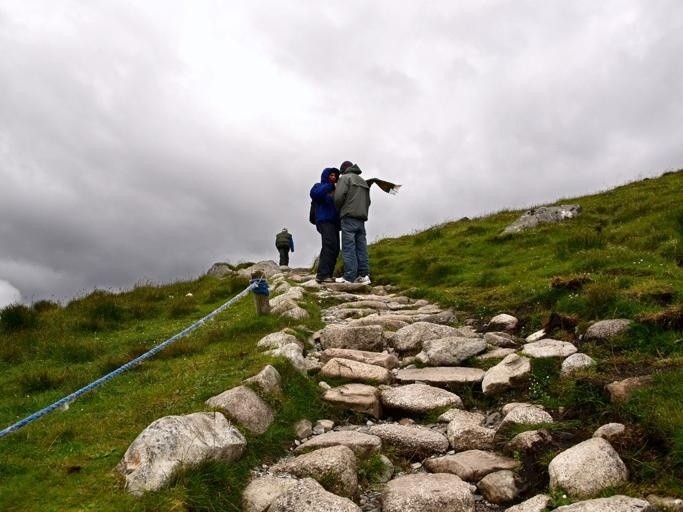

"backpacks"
<box><xmin>309</xmin><ymin>198</ymin><xmax>316</xmax><ymax>224</ymax></box>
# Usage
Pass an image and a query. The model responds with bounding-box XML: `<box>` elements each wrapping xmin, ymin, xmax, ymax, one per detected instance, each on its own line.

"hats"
<box><xmin>338</xmin><ymin>160</ymin><xmax>353</xmax><ymax>173</ymax></box>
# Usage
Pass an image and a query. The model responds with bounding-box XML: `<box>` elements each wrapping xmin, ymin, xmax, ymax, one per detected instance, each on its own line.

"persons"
<box><xmin>275</xmin><ymin>227</ymin><xmax>294</xmax><ymax>267</ymax></box>
<box><xmin>334</xmin><ymin>161</ymin><xmax>371</xmax><ymax>283</ymax></box>
<box><xmin>309</xmin><ymin>167</ymin><xmax>339</xmax><ymax>282</ymax></box>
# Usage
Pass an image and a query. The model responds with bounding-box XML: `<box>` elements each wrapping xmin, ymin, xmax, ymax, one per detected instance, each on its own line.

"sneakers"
<box><xmin>315</xmin><ymin>276</ymin><xmax>336</xmax><ymax>282</ymax></box>
<box><xmin>334</xmin><ymin>276</ymin><xmax>352</xmax><ymax>283</ymax></box>
<box><xmin>353</xmin><ymin>274</ymin><xmax>372</xmax><ymax>285</ymax></box>
